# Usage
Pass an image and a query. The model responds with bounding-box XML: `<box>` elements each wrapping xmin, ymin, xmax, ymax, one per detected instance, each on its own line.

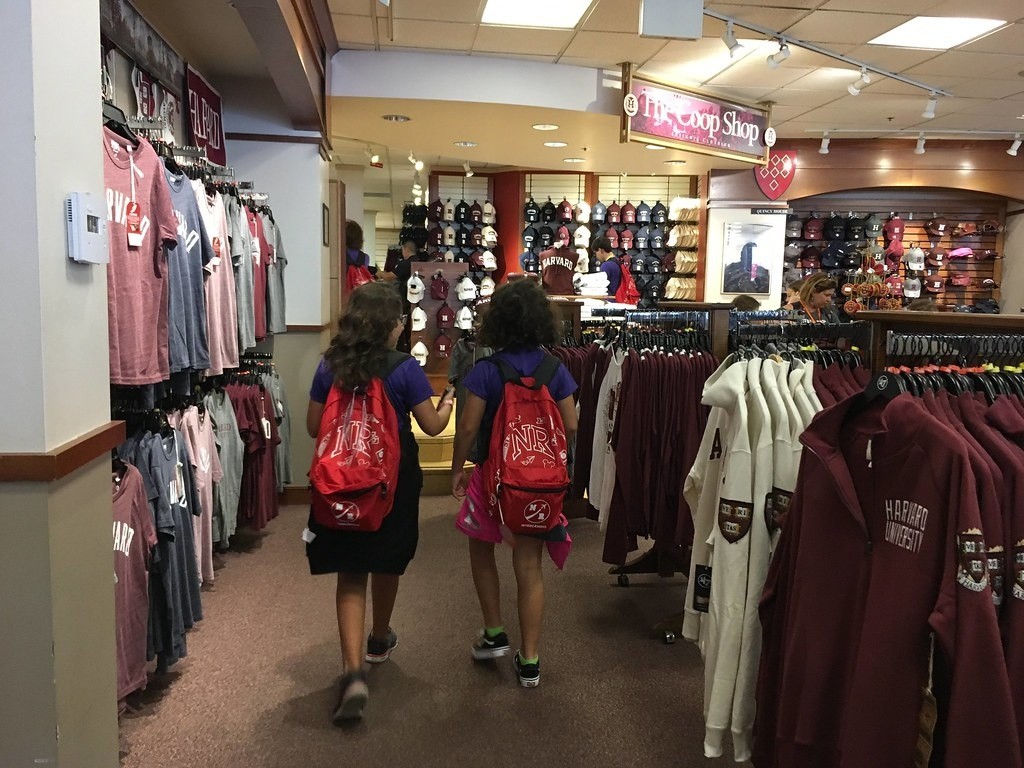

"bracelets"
<box><xmin>440</xmin><ymin>400</ymin><xmax>453</xmax><ymax>411</ymax></box>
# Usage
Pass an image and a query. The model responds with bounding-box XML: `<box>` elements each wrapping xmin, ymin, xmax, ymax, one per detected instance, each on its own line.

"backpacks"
<box><xmin>474</xmin><ymin>355</ymin><xmax>570</xmax><ymax>531</ymax></box>
<box><xmin>606</xmin><ymin>259</ymin><xmax>640</xmax><ymax>305</ymax></box>
<box><xmin>309</xmin><ymin>351</ymin><xmax>415</xmax><ymax>531</ymax></box>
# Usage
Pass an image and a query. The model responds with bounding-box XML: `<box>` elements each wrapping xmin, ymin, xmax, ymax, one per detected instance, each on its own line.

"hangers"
<box><xmin>537</xmin><ymin>309</ymin><xmax>1024</xmax><ymax>419</ymax></box>
<box><xmin>112</xmin><ymin>352</ymin><xmax>280</xmax><ymax>475</ymax></box>
<box><xmin>101</xmin><ymin>69</ymin><xmax>275</xmax><ymax>225</ymax></box>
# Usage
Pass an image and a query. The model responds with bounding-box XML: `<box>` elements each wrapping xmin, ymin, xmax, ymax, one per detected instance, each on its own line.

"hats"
<box><xmin>781</xmin><ymin>215</ymin><xmax>1002</xmax><ymax>315</ymax></box>
<box><xmin>520</xmin><ymin>197</ymin><xmax>667</xmax><ymax>297</ymax></box>
<box><xmin>407</xmin><ymin>198</ymin><xmax>497</xmax><ymax>367</ymax></box>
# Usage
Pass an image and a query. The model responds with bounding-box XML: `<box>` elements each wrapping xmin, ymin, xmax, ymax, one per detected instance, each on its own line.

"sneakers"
<box><xmin>331</xmin><ymin>672</ymin><xmax>369</xmax><ymax>724</ymax></box>
<box><xmin>513</xmin><ymin>647</ymin><xmax>540</xmax><ymax>688</ymax></box>
<box><xmin>366</xmin><ymin>626</ymin><xmax>398</xmax><ymax>662</ymax></box>
<box><xmin>471</xmin><ymin>629</ymin><xmax>511</xmax><ymax>659</ymax></box>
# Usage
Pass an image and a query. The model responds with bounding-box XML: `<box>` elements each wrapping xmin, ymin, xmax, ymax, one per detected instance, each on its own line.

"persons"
<box><xmin>731</xmin><ymin>295</ymin><xmax>759</xmax><ymax>312</ymax></box>
<box><xmin>787</xmin><ymin>280</ymin><xmax>805</xmax><ymax>303</ymax></box>
<box><xmin>591</xmin><ymin>236</ymin><xmax>621</xmax><ymax>302</ymax></box>
<box><xmin>783</xmin><ymin>272</ymin><xmax>839</xmax><ymax>323</ymax></box>
<box><xmin>539</xmin><ymin>242</ymin><xmax>579</xmax><ymax>294</ymax></box>
<box><xmin>306</xmin><ymin>281</ymin><xmax>454</xmax><ymax>724</ymax></box>
<box><xmin>345</xmin><ymin>220</ymin><xmax>369</xmax><ymax>272</ymax></box>
<box><xmin>375</xmin><ymin>241</ymin><xmax>419</xmax><ymax>354</ymax></box>
<box><xmin>907</xmin><ymin>299</ymin><xmax>938</xmax><ymax>311</ymax></box>
<box><xmin>449</xmin><ymin>280</ymin><xmax>579</xmax><ymax>689</ymax></box>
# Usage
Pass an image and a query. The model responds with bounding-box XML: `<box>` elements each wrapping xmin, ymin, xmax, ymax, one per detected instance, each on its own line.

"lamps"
<box><xmin>722</xmin><ymin>20</ymin><xmax>743</xmax><ymax>59</ymax></box>
<box><xmin>922</xmin><ymin>90</ymin><xmax>937</xmax><ymax>118</ymax></box>
<box><xmin>407</xmin><ymin>150</ymin><xmax>424</xmax><ymax>190</ymax></box>
<box><xmin>364</xmin><ymin>144</ymin><xmax>379</xmax><ymax>163</ymax></box>
<box><xmin>915</xmin><ymin>131</ymin><xmax>926</xmax><ymax>154</ymax></box>
<box><xmin>1006</xmin><ymin>133</ymin><xmax>1023</xmax><ymax>156</ymax></box>
<box><xmin>818</xmin><ymin>130</ymin><xmax>830</xmax><ymax>155</ymax></box>
<box><xmin>463</xmin><ymin>161</ymin><xmax>474</xmax><ymax>179</ymax></box>
<box><xmin>848</xmin><ymin>67</ymin><xmax>870</xmax><ymax>96</ymax></box>
<box><xmin>766</xmin><ymin>38</ymin><xmax>791</xmax><ymax>68</ymax></box>
